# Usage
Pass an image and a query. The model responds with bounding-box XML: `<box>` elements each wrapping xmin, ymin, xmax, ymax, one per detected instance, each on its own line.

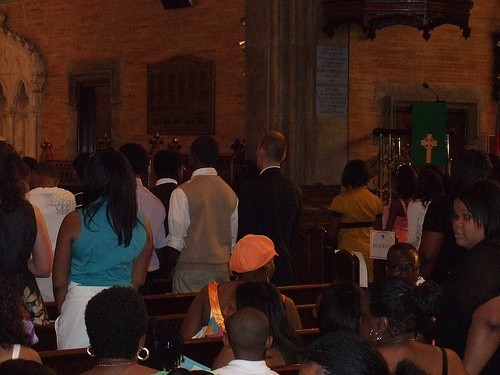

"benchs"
<box><xmin>29</xmin><ymin>280</ymin><xmax>331</xmax><ymax>375</ymax></box>
